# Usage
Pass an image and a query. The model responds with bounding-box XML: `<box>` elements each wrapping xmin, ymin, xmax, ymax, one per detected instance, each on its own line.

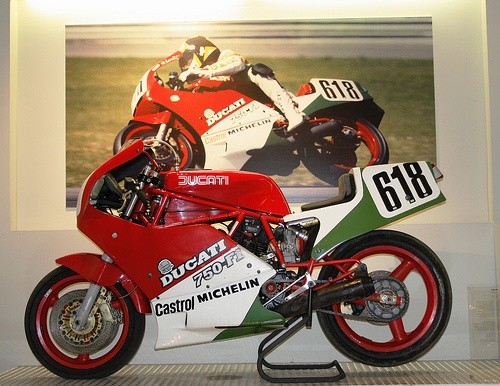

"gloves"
<box><xmin>178</xmin><ymin>67</ymin><xmax>211</xmax><ymax>82</ymax></box>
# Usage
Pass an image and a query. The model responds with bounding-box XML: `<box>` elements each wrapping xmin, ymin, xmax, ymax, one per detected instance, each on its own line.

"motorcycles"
<box><xmin>113</xmin><ymin>50</ymin><xmax>389</xmax><ymax>188</ymax></box>
<box><xmin>23</xmin><ymin>141</ymin><xmax>453</xmax><ymax>384</ymax></box>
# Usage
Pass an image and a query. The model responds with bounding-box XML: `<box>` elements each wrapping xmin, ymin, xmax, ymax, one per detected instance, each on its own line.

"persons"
<box><xmin>177</xmin><ymin>35</ymin><xmax>310</xmax><ymax>133</ymax></box>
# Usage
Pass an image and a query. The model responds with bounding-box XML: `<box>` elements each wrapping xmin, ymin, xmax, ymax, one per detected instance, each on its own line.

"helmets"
<box><xmin>178</xmin><ymin>36</ymin><xmax>220</xmax><ymax>70</ymax></box>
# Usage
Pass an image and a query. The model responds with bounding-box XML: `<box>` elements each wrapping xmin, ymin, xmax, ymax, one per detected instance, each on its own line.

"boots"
<box><xmin>247</xmin><ymin>65</ymin><xmax>310</xmax><ymax>133</ymax></box>
